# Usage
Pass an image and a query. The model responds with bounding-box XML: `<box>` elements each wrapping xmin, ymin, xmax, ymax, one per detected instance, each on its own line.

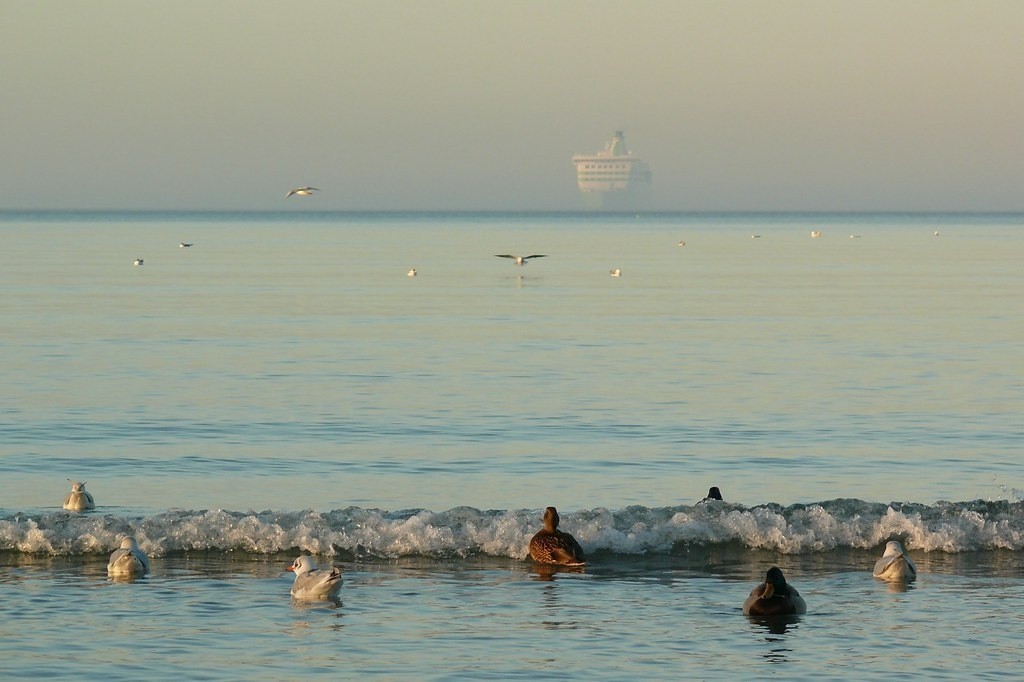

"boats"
<box><xmin>573</xmin><ymin>128</ymin><xmax>646</xmax><ymax>191</ymax></box>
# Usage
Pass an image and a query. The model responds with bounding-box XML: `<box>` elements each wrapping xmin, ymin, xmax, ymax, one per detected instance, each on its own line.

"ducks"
<box><xmin>742</xmin><ymin>567</ymin><xmax>807</xmax><ymax>615</ymax></box>
<box><xmin>106</xmin><ymin>536</ymin><xmax>149</xmax><ymax>573</ymax></box>
<box><xmin>284</xmin><ymin>555</ymin><xmax>344</xmax><ymax>597</ymax></box>
<box><xmin>873</xmin><ymin>541</ymin><xmax>916</xmax><ymax>579</ymax></box>
<box><xmin>696</xmin><ymin>487</ymin><xmax>723</xmax><ymax>506</ymax></box>
<box><xmin>529</xmin><ymin>506</ymin><xmax>587</xmax><ymax>567</ymax></box>
<box><xmin>62</xmin><ymin>482</ymin><xmax>95</xmax><ymax>511</ymax></box>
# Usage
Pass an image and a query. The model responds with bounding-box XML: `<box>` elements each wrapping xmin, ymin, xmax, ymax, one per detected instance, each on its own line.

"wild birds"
<box><xmin>179</xmin><ymin>242</ymin><xmax>194</xmax><ymax>247</ymax></box>
<box><xmin>133</xmin><ymin>258</ymin><xmax>143</xmax><ymax>265</ymax></box>
<box><xmin>494</xmin><ymin>254</ymin><xmax>549</xmax><ymax>266</ymax></box>
<box><xmin>677</xmin><ymin>230</ymin><xmax>941</xmax><ymax>247</ymax></box>
<box><xmin>284</xmin><ymin>187</ymin><xmax>319</xmax><ymax>200</ymax></box>
<box><xmin>610</xmin><ymin>268</ymin><xmax>621</xmax><ymax>277</ymax></box>
<box><xmin>407</xmin><ymin>269</ymin><xmax>417</xmax><ymax>277</ymax></box>
